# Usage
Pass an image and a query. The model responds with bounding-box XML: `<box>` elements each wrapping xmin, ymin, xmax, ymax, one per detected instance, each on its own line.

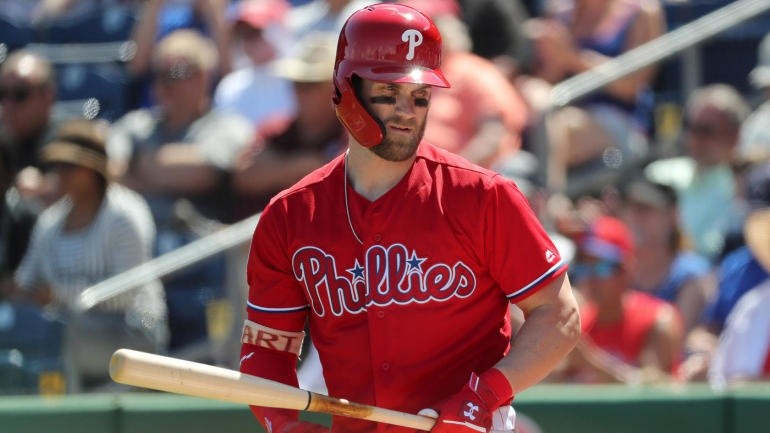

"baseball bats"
<box><xmin>108</xmin><ymin>348</ymin><xmax>436</xmax><ymax>433</ymax></box>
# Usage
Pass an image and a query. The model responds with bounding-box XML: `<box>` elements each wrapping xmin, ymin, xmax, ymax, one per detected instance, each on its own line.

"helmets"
<box><xmin>331</xmin><ymin>3</ymin><xmax>451</xmax><ymax>148</ymax></box>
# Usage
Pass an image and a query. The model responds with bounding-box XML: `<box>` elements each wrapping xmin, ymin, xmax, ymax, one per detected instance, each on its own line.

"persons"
<box><xmin>240</xmin><ymin>2</ymin><xmax>582</xmax><ymax>433</ymax></box>
<box><xmin>0</xmin><ymin>0</ymin><xmax>770</xmax><ymax>388</ymax></box>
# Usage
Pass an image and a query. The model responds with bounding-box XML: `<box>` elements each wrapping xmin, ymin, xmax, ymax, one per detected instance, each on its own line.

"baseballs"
<box><xmin>417</xmin><ymin>408</ymin><xmax>438</xmax><ymax>418</ymax></box>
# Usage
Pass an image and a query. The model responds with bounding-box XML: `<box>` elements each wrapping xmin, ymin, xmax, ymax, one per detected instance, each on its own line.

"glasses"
<box><xmin>680</xmin><ymin>114</ymin><xmax>735</xmax><ymax>141</ymax></box>
<box><xmin>0</xmin><ymin>80</ymin><xmax>51</xmax><ymax>105</ymax></box>
<box><xmin>566</xmin><ymin>260</ymin><xmax>623</xmax><ymax>283</ymax></box>
<box><xmin>744</xmin><ymin>179</ymin><xmax>769</xmax><ymax>208</ymax></box>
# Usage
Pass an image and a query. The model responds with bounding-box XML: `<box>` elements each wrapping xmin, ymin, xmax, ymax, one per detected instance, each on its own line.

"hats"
<box><xmin>227</xmin><ymin>0</ymin><xmax>291</xmax><ymax>41</ymax></box>
<box><xmin>567</xmin><ymin>212</ymin><xmax>635</xmax><ymax>268</ymax></box>
<box><xmin>39</xmin><ymin>120</ymin><xmax>109</xmax><ymax>179</ymax></box>
<box><xmin>273</xmin><ymin>29</ymin><xmax>339</xmax><ymax>82</ymax></box>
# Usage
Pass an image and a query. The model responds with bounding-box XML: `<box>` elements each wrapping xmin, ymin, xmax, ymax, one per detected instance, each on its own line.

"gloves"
<box><xmin>271</xmin><ymin>419</ymin><xmax>332</xmax><ymax>433</ymax></box>
<box><xmin>427</xmin><ymin>368</ymin><xmax>513</xmax><ymax>433</ymax></box>
